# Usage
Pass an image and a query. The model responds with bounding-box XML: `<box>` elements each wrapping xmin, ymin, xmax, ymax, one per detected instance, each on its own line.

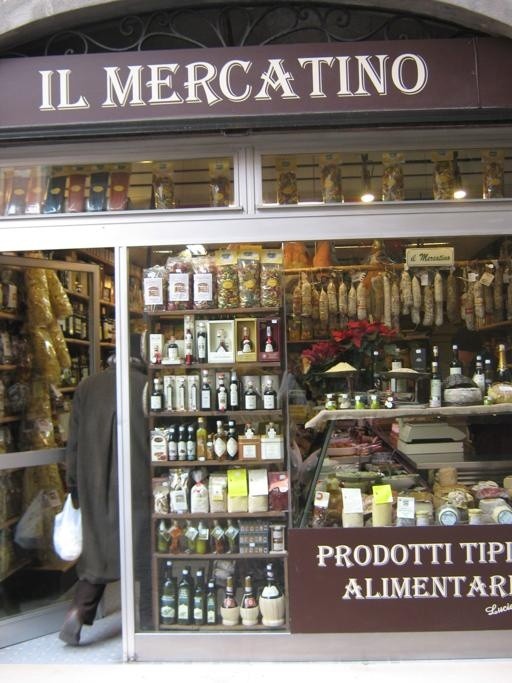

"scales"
<box><xmin>398</xmin><ymin>423</ymin><xmax>468</xmax><ymax>444</ymax></box>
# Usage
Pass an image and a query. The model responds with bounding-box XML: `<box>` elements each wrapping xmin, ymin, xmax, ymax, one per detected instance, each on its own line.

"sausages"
<box><xmin>447</xmin><ymin>273</ymin><xmax>456</xmax><ymax>321</ymax></box>
<box><xmin>400</xmin><ymin>270</ymin><xmax>412</xmax><ymax>315</ymax></box>
<box><xmin>293</xmin><ymin>286</ymin><xmax>302</xmax><ymax>315</ymax></box>
<box><xmin>506</xmin><ymin>274</ymin><xmax>512</xmax><ymax>318</ymax></box>
<box><xmin>328</xmin><ymin>280</ymin><xmax>337</xmax><ymax>314</ymax></box>
<box><xmin>412</xmin><ymin>308</ymin><xmax>421</xmax><ymax>324</ymax></box>
<box><xmin>435</xmin><ymin>272</ymin><xmax>443</xmax><ymax>326</ymax></box>
<box><xmin>349</xmin><ymin>284</ymin><xmax>357</xmax><ymax>318</ymax></box>
<box><xmin>312</xmin><ymin>287</ymin><xmax>320</xmax><ymax>320</ymax></box>
<box><xmin>461</xmin><ymin>292</ymin><xmax>467</xmax><ymax>320</ymax></box>
<box><xmin>383</xmin><ymin>274</ymin><xmax>391</xmax><ymax>330</ymax></box>
<box><xmin>391</xmin><ymin>282</ymin><xmax>400</xmax><ymax>316</ymax></box>
<box><xmin>371</xmin><ymin>276</ymin><xmax>384</xmax><ymax>321</ymax></box>
<box><xmin>412</xmin><ymin>276</ymin><xmax>422</xmax><ymax>308</ymax></box>
<box><xmin>466</xmin><ymin>292</ymin><xmax>475</xmax><ymax>331</ymax></box>
<box><xmin>356</xmin><ymin>280</ymin><xmax>367</xmax><ymax>320</ymax></box>
<box><xmin>423</xmin><ymin>284</ymin><xmax>433</xmax><ymax>326</ymax></box>
<box><xmin>302</xmin><ymin>272</ymin><xmax>312</xmax><ymax>318</ymax></box>
<box><xmin>494</xmin><ymin>268</ymin><xmax>503</xmax><ymax>310</ymax></box>
<box><xmin>484</xmin><ymin>266</ymin><xmax>493</xmax><ymax>314</ymax></box>
<box><xmin>319</xmin><ymin>288</ymin><xmax>329</xmax><ymax>321</ymax></box>
<box><xmin>339</xmin><ymin>280</ymin><xmax>348</xmax><ymax>317</ymax></box>
<box><xmin>474</xmin><ymin>280</ymin><xmax>485</xmax><ymax>318</ymax></box>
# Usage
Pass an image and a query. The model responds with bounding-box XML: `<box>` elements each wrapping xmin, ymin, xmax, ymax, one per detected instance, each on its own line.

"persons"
<box><xmin>59</xmin><ymin>333</ymin><xmax>152</xmax><ymax>646</ymax></box>
<box><xmin>449</xmin><ymin>332</ymin><xmax>512</xmax><ymax>455</ymax></box>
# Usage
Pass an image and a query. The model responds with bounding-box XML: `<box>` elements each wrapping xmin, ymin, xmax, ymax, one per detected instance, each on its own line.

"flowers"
<box><xmin>290</xmin><ymin>319</ymin><xmax>398</xmax><ymax>385</ymax></box>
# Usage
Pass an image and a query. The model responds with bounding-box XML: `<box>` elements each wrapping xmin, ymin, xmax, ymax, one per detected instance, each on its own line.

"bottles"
<box><xmin>62</xmin><ymin>353</ymin><xmax>92</xmax><ymax>386</ymax></box>
<box><xmin>383</xmin><ymin>153</ymin><xmax>404</xmax><ymax>200</ymax></box>
<box><xmin>102</xmin><ymin>305</ymin><xmax>110</xmax><ymax>342</ymax></box>
<box><xmin>177</xmin><ymin>570</ymin><xmax>192</xmax><ymax>623</ymax></box>
<box><xmin>264</xmin><ymin>323</ymin><xmax>277</xmax><ymax>352</ymax></box>
<box><xmin>166</xmin><ymin>377</ymin><xmax>173</xmax><ymax>412</ymax></box>
<box><xmin>167</xmin><ymin>336</ymin><xmax>179</xmax><ymax>359</ymax></box>
<box><xmin>246</xmin><ymin>423</ymin><xmax>255</xmax><ymax>437</ymax></box>
<box><xmin>450</xmin><ymin>344</ymin><xmax>463</xmax><ymax>376</ymax></box>
<box><xmin>89</xmin><ymin>164</ymin><xmax>110</xmax><ymax>212</ymax></box>
<box><xmin>170</xmin><ymin>520</ymin><xmax>179</xmax><ymax>552</ymax></box>
<box><xmin>164</xmin><ymin>559</ymin><xmax>172</xmax><ymax>577</ymax></box>
<box><xmin>475</xmin><ymin>362</ymin><xmax>487</xmax><ymax>396</ymax></box>
<box><xmin>240</xmin><ymin>576</ymin><xmax>261</xmax><ymax>626</ymax></box>
<box><xmin>111</xmin><ymin>164</ymin><xmax>129</xmax><ymax>210</ymax></box>
<box><xmin>485</xmin><ymin>359</ymin><xmax>491</xmax><ymax>387</ymax></box>
<box><xmin>324</xmin><ymin>394</ymin><xmax>336</xmax><ymax>410</ymax></box>
<box><xmin>151</xmin><ymin>436</ymin><xmax>168</xmax><ymax>461</ymax></box>
<box><xmin>194</xmin><ymin>569</ymin><xmax>206</xmax><ymax>625</ymax></box>
<box><xmin>201</xmin><ymin>370</ymin><xmax>212</xmax><ymax>411</ymax></box>
<box><xmin>355</xmin><ymin>395</ymin><xmax>365</xmax><ymax>407</ymax></box>
<box><xmin>178</xmin><ymin>426</ymin><xmax>188</xmax><ymax>460</ymax></box>
<box><xmin>30</xmin><ymin>166</ymin><xmax>44</xmax><ymax>213</ymax></box>
<box><xmin>188</xmin><ymin>426</ymin><xmax>196</xmax><ymax>461</ymax></box>
<box><xmin>213</xmin><ymin>519</ymin><xmax>225</xmax><ymax>553</ymax></box>
<box><xmin>417</xmin><ymin>511</ymin><xmax>429</xmax><ymax>526</ymax></box>
<box><xmin>216</xmin><ymin>373</ymin><xmax>228</xmax><ymax>411</ymax></box>
<box><xmin>432</xmin><ymin>345</ymin><xmax>442</xmax><ymax>376</ymax></box>
<box><xmin>215</xmin><ymin>420</ymin><xmax>227</xmax><ymax>460</ymax></box>
<box><xmin>8</xmin><ymin>168</ymin><xmax>29</xmax><ymax>214</ymax></box>
<box><xmin>387</xmin><ymin>397</ymin><xmax>395</xmax><ymax>408</ymax></box>
<box><xmin>244</xmin><ymin>381</ymin><xmax>257</xmax><ymax>410</ymax></box>
<box><xmin>168</xmin><ymin>428</ymin><xmax>178</xmax><ymax>460</ymax></box>
<box><xmin>216</xmin><ymin>330</ymin><xmax>228</xmax><ymax>353</ymax></box>
<box><xmin>158</xmin><ymin>519</ymin><xmax>167</xmax><ymax>553</ymax></box>
<box><xmin>264</xmin><ymin>379</ymin><xmax>277</xmax><ymax>410</ymax></box>
<box><xmin>197</xmin><ymin>417</ymin><xmax>207</xmax><ymax>461</ymax></box>
<box><xmin>474</xmin><ymin>356</ymin><xmax>482</xmax><ymax>383</ymax></box>
<box><xmin>395</xmin><ymin>509</ymin><xmax>415</xmax><ymax>526</ymax></box>
<box><xmin>151</xmin><ymin>379</ymin><xmax>163</xmax><ymax>411</ymax></box>
<box><xmin>436</xmin><ymin>503</ymin><xmax>461</xmax><ymax>525</ymax></box>
<box><xmin>413</xmin><ymin>347</ymin><xmax>425</xmax><ymax>372</ymax></box>
<box><xmin>260</xmin><ymin>563</ymin><xmax>286</xmax><ymax>626</ymax></box>
<box><xmin>429</xmin><ymin>363</ymin><xmax>441</xmax><ymax>409</ymax></box>
<box><xmin>469</xmin><ymin>509</ymin><xmax>483</xmax><ymax>525</ymax></box>
<box><xmin>1</xmin><ymin>267</ymin><xmax>27</xmax><ymax>314</ymax></box>
<box><xmin>373</xmin><ymin>350</ymin><xmax>381</xmax><ymax>389</ymax></box>
<box><xmin>60</xmin><ymin>297</ymin><xmax>90</xmax><ymax>339</ymax></box>
<box><xmin>151</xmin><ymin>163</ymin><xmax>175</xmax><ymax>209</ymax></box>
<box><xmin>338</xmin><ymin>394</ymin><xmax>350</xmax><ymax>407</ymax></box>
<box><xmin>313</xmin><ymin>500</ymin><xmax>327</xmax><ymax>528</ymax></box>
<box><xmin>207</xmin><ymin>165</ymin><xmax>229</xmax><ymax>207</ymax></box>
<box><xmin>207</xmin><ymin>434</ymin><xmax>213</xmax><ymax>459</ymax></box>
<box><xmin>109</xmin><ymin>308</ymin><xmax>114</xmax><ymax>342</ymax></box>
<box><xmin>0</xmin><ymin>321</ymin><xmax>21</xmax><ymax>364</ymax></box>
<box><xmin>199</xmin><ymin>567</ymin><xmax>206</xmax><ymax>585</ymax></box>
<box><xmin>372</xmin><ymin>497</ymin><xmax>392</xmax><ymax>527</ymax></box>
<box><xmin>185</xmin><ymin>316</ymin><xmax>193</xmax><ymax>365</ymax></box>
<box><xmin>322</xmin><ymin>155</ymin><xmax>344</xmax><ymax>204</ymax></box>
<box><xmin>151</xmin><ymin>346</ymin><xmax>160</xmax><ymax>365</ymax></box>
<box><xmin>241</xmin><ymin>327</ymin><xmax>253</xmax><ymax>353</ymax></box>
<box><xmin>497</xmin><ymin>344</ymin><xmax>510</xmax><ymax>383</ymax></box>
<box><xmin>44</xmin><ymin>166</ymin><xmax>65</xmax><ymax>213</ymax></box>
<box><xmin>221</xmin><ymin>576</ymin><xmax>241</xmax><ymax>626</ymax></box>
<box><xmin>392</xmin><ymin>348</ymin><xmax>404</xmax><ymax>393</ymax></box>
<box><xmin>186</xmin><ymin>565</ymin><xmax>195</xmax><ymax>587</ymax></box>
<box><xmin>227</xmin><ymin>420</ymin><xmax>239</xmax><ymax>460</ymax></box>
<box><xmin>230</xmin><ymin>368</ymin><xmax>241</xmax><ymax>410</ymax></box>
<box><xmin>277</xmin><ymin>162</ymin><xmax>297</xmax><ymax>203</ymax></box>
<box><xmin>432</xmin><ymin>152</ymin><xmax>454</xmax><ymax>198</ymax></box>
<box><xmin>197</xmin><ymin>518</ymin><xmax>206</xmax><ymax>554</ymax></box>
<box><xmin>385</xmin><ymin>399</ymin><xmax>388</xmax><ymax>407</ymax></box>
<box><xmin>184</xmin><ymin>520</ymin><xmax>196</xmax><ymax>550</ymax></box>
<box><xmin>160</xmin><ymin>571</ymin><xmax>177</xmax><ymax>624</ymax></box>
<box><xmin>342</xmin><ymin>499</ymin><xmax>363</xmax><ymax>527</ymax></box>
<box><xmin>483</xmin><ymin>151</ymin><xmax>503</xmax><ymax>198</ymax></box>
<box><xmin>179</xmin><ymin>378</ymin><xmax>185</xmax><ymax>411</ymax></box>
<box><xmin>273</xmin><ymin>526</ymin><xmax>284</xmax><ymax>550</ymax></box>
<box><xmin>206</xmin><ymin>578</ymin><xmax>217</xmax><ymax>623</ymax></box>
<box><xmin>371</xmin><ymin>395</ymin><xmax>381</xmax><ymax>409</ymax></box>
<box><xmin>493</xmin><ymin>505</ymin><xmax>512</xmax><ymax>524</ymax></box>
<box><xmin>227</xmin><ymin>520</ymin><xmax>237</xmax><ymax>552</ymax></box>
<box><xmin>197</xmin><ymin>323</ymin><xmax>207</xmax><ymax>362</ymax></box>
<box><xmin>268</xmin><ymin>422</ymin><xmax>276</xmax><ymax>438</ymax></box>
<box><xmin>65</xmin><ymin>165</ymin><xmax>85</xmax><ymax>213</ymax></box>
<box><xmin>191</xmin><ymin>376</ymin><xmax>197</xmax><ymax>411</ymax></box>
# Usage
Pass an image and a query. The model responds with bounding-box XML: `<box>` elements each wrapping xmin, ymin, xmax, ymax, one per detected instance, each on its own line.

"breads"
<box><xmin>489</xmin><ymin>380</ymin><xmax>512</xmax><ymax>402</ymax></box>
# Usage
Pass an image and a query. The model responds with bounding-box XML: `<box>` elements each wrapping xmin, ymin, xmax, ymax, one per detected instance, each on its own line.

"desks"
<box><xmin>399</xmin><ymin>453</ymin><xmax>511</xmax><ymax>486</ymax></box>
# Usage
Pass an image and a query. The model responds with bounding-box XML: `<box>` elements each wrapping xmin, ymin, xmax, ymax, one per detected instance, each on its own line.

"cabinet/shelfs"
<box><xmin>0</xmin><ymin>248</ymin><xmax>144</xmax><ymax>585</ymax></box>
<box><xmin>281</xmin><ymin>240</ymin><xmax>512</xmax><ymax>415</ymax></box>
<box><xmin>290</xmin><ymin>418</ymin><xmax>512</xmax><ymax>630</ymax></box>
<box><xmin>127</xmin><ymin>244</ymin><xmax>287</xmax><ymax>633</ymax></box>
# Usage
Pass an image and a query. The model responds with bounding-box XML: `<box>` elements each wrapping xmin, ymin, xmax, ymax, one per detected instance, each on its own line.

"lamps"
<box><xmin>452</xmin><ymin>152</ymin><xmax>467</xmax><ymax>199</ymax></box>
<box><xmin>360</xmin><ymin>153</ymin><xmax>375</xmax><ymax>203</ymax></box>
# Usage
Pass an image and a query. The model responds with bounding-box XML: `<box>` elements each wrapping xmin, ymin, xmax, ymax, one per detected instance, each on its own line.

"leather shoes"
<box><xmin>59</xmin><ymin>609</ymin><xmax>83</xmax><ymax>647</ymax></box>
